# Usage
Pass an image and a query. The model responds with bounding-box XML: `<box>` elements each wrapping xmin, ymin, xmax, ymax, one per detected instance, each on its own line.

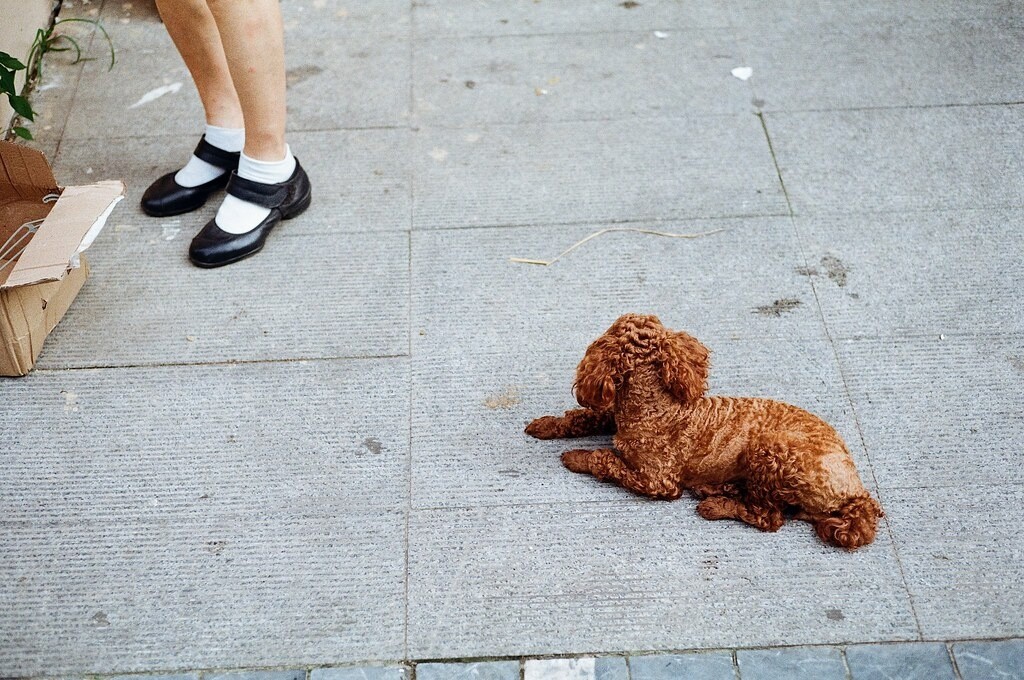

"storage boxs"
<box><xmin>0</xmin><ymin>138</ymin><xmax>127</xmax><ymax>379</ymax></box>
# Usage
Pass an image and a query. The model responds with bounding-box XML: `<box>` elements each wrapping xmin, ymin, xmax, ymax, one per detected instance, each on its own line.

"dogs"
<box><xmin>523</xmin><ymin>312</ymin><xmax>886</xmax><ymax>553</ymax></box>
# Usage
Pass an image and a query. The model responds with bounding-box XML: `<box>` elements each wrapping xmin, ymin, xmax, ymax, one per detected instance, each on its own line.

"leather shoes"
<box><xmin>141</xmin><ymin>133</ymin><xmax>239</xmax><ymax>217</ymax></box>
<box><xmin>189</xmin><ymin>156</ymin><xmax>312</xmax><ymax>267</ymax></box>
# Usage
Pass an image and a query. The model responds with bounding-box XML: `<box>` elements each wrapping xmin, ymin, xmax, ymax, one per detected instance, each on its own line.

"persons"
<box><xmin>140</xmin><ymin>0</ymin><xmax>313</xmax><ymax>270</ymax></box>
